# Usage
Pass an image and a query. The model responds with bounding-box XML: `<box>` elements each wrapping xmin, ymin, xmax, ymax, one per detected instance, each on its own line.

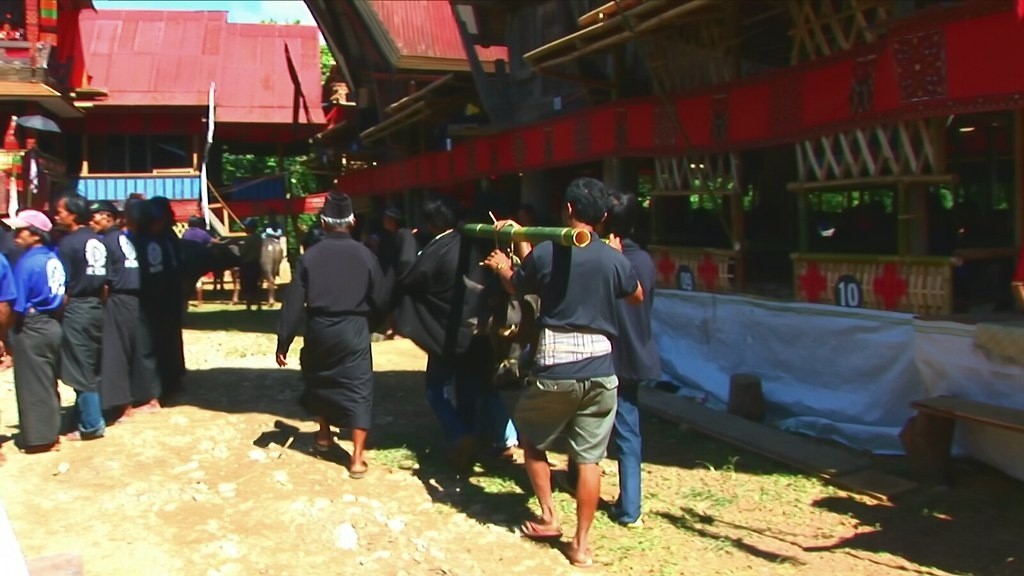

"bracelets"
<box><xmin>494</xmin><ymin>262</ymin><xmax>510</xmax><ymax>271</ymax></box>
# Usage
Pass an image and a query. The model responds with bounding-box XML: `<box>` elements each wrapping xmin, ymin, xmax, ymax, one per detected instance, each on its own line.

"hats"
<box><xmin>320</xmin><ymin>193</ymin><xmax>354</xmax><ymax>223</ymax></box>
<box><xmin>241</xmin><ymin>218</ymin><xmax>259</xmax><ymax>226</ymax></box>
<box><xmin>2</xmin><ymin>210</ymin><xmax>53</xmax><ymax>232</ymax></box>
<box><xmin>88</xmin><ymin>200</ymin><xmax>120</xmax><ymax>214</ymax></box>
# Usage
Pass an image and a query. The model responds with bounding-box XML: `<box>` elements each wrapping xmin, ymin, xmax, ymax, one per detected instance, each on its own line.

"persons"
<box><xmin>276</xmin><ymin>178</ymin><xmax>658</xmax><ymax>568</ymax></box>
<box><xmin>182</xmin><ymin>215</ymin><xmax>283</xmax><ymax>307</ymax></box>
<box><xmin>0</xmin><ymin>192</ymin><xmax>185</xmax><ymax>467</ymax></box>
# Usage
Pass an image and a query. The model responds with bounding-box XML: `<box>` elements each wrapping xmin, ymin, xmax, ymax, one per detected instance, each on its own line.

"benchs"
<box><xmin>901</xmin><ymin>395</ymin><xmax>1024</xmax><ymax>486</ymax></box>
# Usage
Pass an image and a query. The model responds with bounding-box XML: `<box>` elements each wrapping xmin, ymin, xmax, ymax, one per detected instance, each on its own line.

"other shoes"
<box><xmin>556</xmin><ymin>473</ymin><xmax>577</xmax><ymax>493</ymax></box>
<box><xmin>135</xmin><ymin>404</ymin><xmax>162</xmax><ymax>414</ymax></box>
<box><xmin>444</xmin><ymin>445</ymin><xmax>485</xmax><ymax>463</ymax></box>
<box><xmin>608</xmin><ymin>501</ymin><xmax>643</xmax><ymax>528</ymax></box>
<box><xmin>106</xmin><ymin>409</ymin><xmax>136</xmax><ymax>422</ymax></box>
<box><xmin>485</xmin><ymin>447</ymin><xmax>519</xmax><ymax>462</ymax></box>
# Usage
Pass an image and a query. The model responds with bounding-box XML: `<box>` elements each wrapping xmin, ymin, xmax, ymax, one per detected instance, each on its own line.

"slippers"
<box><xmin>310</xmin><ymin>431</ymin><xmax>331</xmax><ymax>451</ymax></box>
<box><xmin>349</xmin><ymin>459</ymin><xmax>369</xmax><ymax>479</ymax></box>
<box><xmin>562</xmin><ymin>540</ymin><xmax>592</xmax><ymax>568</ymax></box>
<box><xmin>521</xmin><ymin>515</ymin><xmax>563</xmax><ymax>536</ymax></box>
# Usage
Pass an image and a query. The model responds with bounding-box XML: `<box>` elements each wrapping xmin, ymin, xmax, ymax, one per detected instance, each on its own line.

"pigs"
<box><xmin>462</xmin><ymin>265</ymin><xmax>541</xmax><ymax>381</ymax></box>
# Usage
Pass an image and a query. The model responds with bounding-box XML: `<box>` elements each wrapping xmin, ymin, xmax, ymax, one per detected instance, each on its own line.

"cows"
<box><xmin>208</xmin><ymin>236</ymin><xmax>283</xmax><ymax>308</ymax></box>
